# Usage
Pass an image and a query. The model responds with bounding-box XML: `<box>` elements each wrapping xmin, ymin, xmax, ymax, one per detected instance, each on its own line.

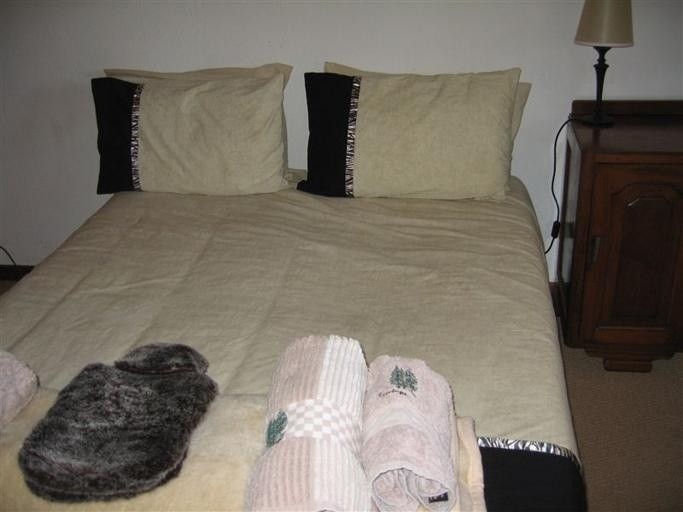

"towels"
<box><xmin>249</xmin><ymin>334</ymin><xmax>457</xmax><ymax>511</ymax></box>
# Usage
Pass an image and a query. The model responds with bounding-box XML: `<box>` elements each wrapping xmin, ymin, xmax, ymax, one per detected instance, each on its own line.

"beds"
<box><xmin>0</xmin><ymin>171</ymin><xmax>587</xmax><ymax>512</ymax></box>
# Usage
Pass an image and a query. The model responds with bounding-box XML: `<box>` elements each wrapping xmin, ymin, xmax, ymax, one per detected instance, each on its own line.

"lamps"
<box><xmin>571</xmin><ymin>0</ymin><xmax>631</xmax><ymax>132</ymax></box>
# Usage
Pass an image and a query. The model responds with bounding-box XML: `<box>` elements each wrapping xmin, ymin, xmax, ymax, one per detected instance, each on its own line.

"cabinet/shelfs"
<box><xmin>554</xmin><ymin>117</ymin><xmax>683</xmax><ymax>376</ymax></box>
